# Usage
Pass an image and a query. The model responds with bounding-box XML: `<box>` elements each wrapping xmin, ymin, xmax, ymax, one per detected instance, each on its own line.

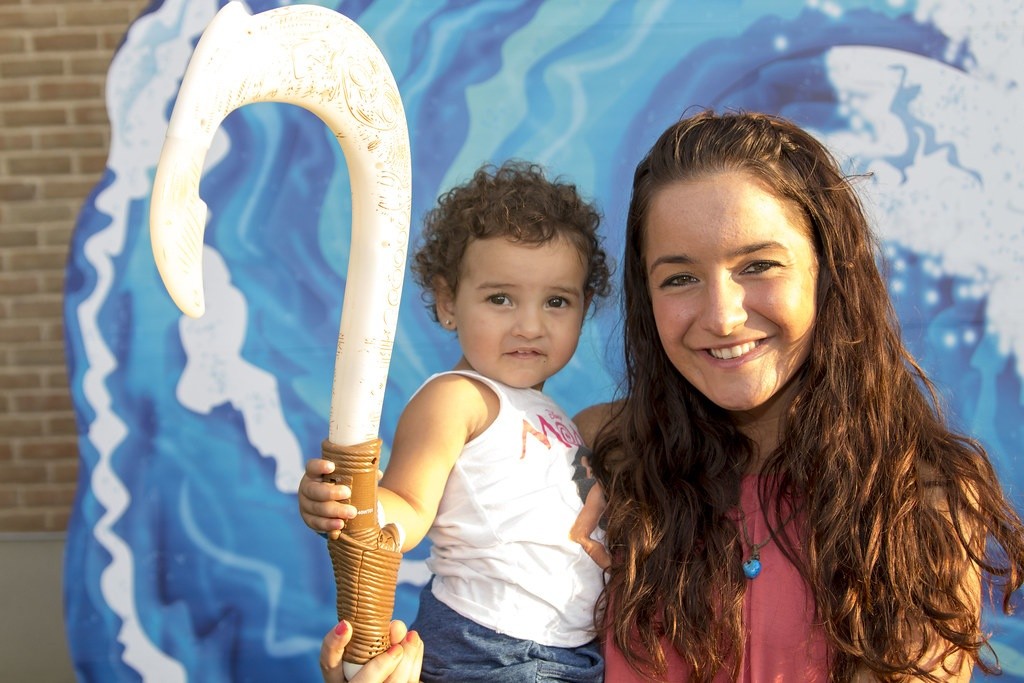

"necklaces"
<box><xmin>739</xmin><ymin>499</ymin><xmax>804</xmax><ymax>579</ymax></box>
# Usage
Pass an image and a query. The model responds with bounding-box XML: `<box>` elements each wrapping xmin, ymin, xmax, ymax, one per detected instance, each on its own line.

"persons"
<box><xmin>299</xmin><ymin>158</ymin><xmax>616</xmax><ymax>683</ymax></box>
<box><xmin>321</xmin><ymin>104</ymin><xmax>1024</xmax><ymax>682</ymax></box>
